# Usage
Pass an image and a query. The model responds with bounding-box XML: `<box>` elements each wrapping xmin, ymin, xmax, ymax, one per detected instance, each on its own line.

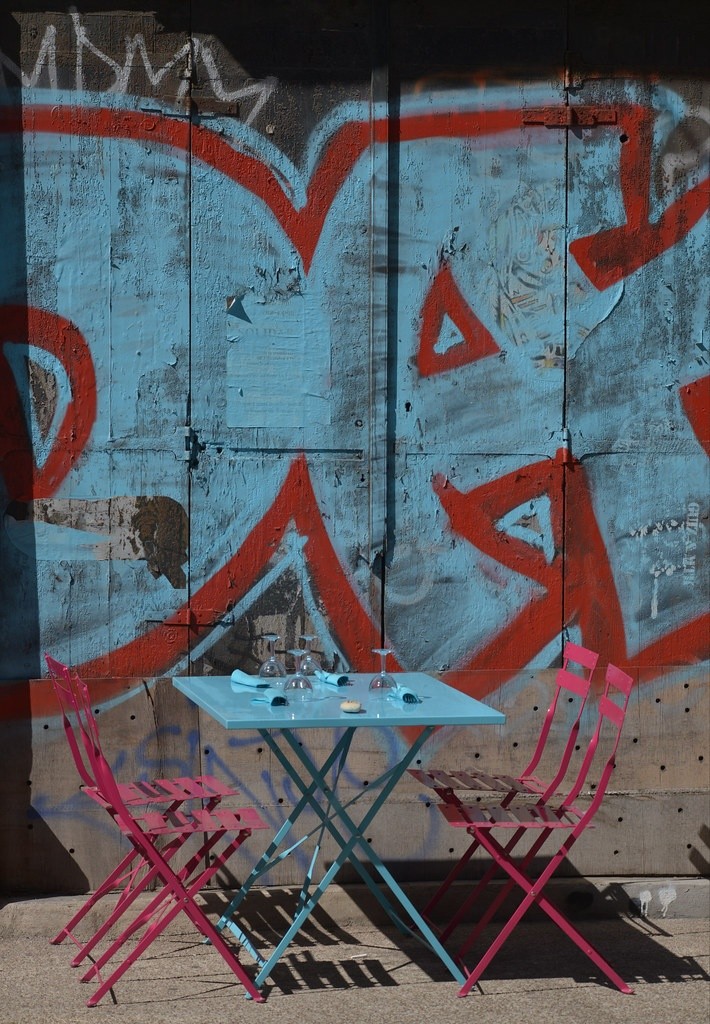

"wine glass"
<box><xmin>259</xmin><ymin>634</ymin><xmax>287</xmax><ymax>688</ymax></box>
<box><xmin>297</xmin><ymin>634</ymin><xmax>322</xmax><ymax>684</ymax></box>
<box><xmin>283</xmin><ymin>649</ymin><xmax>314</xmax><ymax>702</ymax></box>
<box><xmin>368</xmin><ymin>648</ymin><xmax>399</xmax><ymax>704</ymax></box>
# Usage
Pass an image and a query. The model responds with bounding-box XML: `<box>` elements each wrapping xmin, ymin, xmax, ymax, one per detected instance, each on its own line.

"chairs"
<box><xmin>43</xmin><ymin>651</ymin><xmax>243</xmax><ymax>968</ymax></box>
<box><xmin>421</xmin><ymin>641</ymin><xmax>601</xmax><ymax>945</ymax></box>
<box><xmin>445</xmin><ymin>662</ymin><xmax>633</xmax><ymax>998</ymax></box>
<box><xmin>71</xmin><ymin>669</ymin><xmax>267</xmax><ymax>1007</ymax></box>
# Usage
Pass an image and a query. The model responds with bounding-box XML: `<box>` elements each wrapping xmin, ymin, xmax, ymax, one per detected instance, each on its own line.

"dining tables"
<box><xmin>172</xmin><ymin>672</ymin><xmax>479</xmax><ymax>999</ymax></box>
